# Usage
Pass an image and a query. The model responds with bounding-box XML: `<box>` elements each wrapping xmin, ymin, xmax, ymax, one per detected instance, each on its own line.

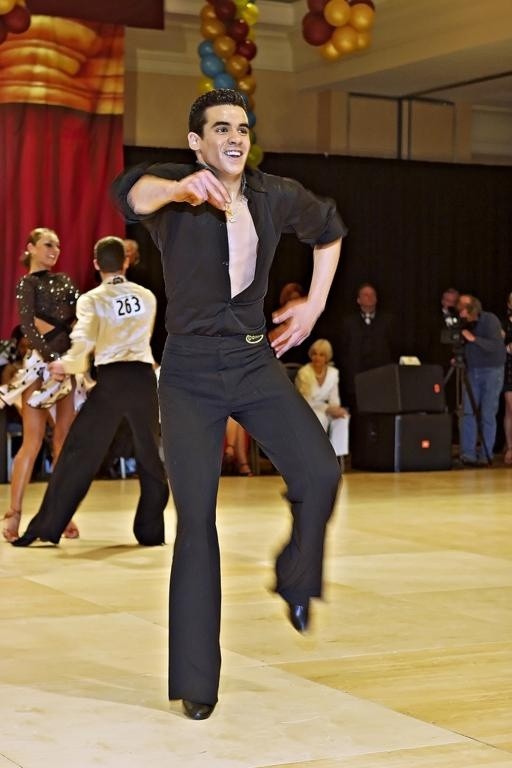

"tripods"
<box><xmin>443</xmin><ymin>348</ymin><xmax>493</xmax><ymax>468</ymax></box>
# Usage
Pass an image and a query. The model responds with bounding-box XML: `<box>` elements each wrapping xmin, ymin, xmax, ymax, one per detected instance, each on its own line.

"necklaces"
<box><xmin>223</xmin><ymin>172</ymin><xmax>247</xmax><ymax>223</ymax></box>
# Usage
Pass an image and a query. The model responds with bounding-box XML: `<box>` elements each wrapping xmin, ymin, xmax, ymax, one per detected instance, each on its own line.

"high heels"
<box><xmin>65</xmin><ymin>521</ymin><xmax>78</xmax><ymax>538</ymax></box>
<box><xmin>0</xmin><ymin>507</ymin><xmax>20</xmax><ymax>543</ymax></box>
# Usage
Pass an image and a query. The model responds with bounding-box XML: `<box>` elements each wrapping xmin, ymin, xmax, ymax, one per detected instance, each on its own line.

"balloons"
<box><xmin>299</xmin><ymin>1</ymin><xmax>377</xmax><ymax>63</ymax></box>
<box><xmin>195</xmin><ymin>0</ymin><xmax>265</xmax><ymax>168</ymax></box>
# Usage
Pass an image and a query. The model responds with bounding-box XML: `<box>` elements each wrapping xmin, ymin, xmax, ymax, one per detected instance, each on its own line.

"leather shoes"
<box><xmin>276</xmin><ymin>556</ymin><xmax>309</xmax><ymax>629</ymax></box>
<box><xmin>12</xmin><ymin>532</ymin><xmax>39</xmax><ymax>547</ymax></box>
<box><xmin>182</xmin><ymin>699</ymin><xmax>212</xmax><ymax>718</ymax></box>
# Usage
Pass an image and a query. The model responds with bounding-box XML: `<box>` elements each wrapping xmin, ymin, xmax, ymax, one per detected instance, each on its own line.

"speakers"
<box><xmin>354</xmin><ymin>364</ymin><xmax>446</xmax><ymax>413</ymax></box>
<box><xmin>352</xmin><ymin>412</ymin><xmax>453</xmax><ymax>472</ymax></box>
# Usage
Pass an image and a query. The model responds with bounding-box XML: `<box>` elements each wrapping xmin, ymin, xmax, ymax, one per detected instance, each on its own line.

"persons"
<box><xmin>8</xmin><ymin>234</ymin><xmax>170</xmax><ymax>548</ymax></box>
<box><xmin>282</xmin><ymin>282</ymin><xmax>512</xmax><ymax>465</ymax></box>
<box><xmin>106</xmin><ymin>85</ymin><xmax>352</xmax><ymax>722</ymax></box>
<box><xmin>1</xmin><ymin>322</ymin><xmax>253</xmax><ymax>477</ymax></box>
<box><xmin>0</xmin><ymin>227</ymin><xmax>95</xmax><ymax>545</ymax></box>
